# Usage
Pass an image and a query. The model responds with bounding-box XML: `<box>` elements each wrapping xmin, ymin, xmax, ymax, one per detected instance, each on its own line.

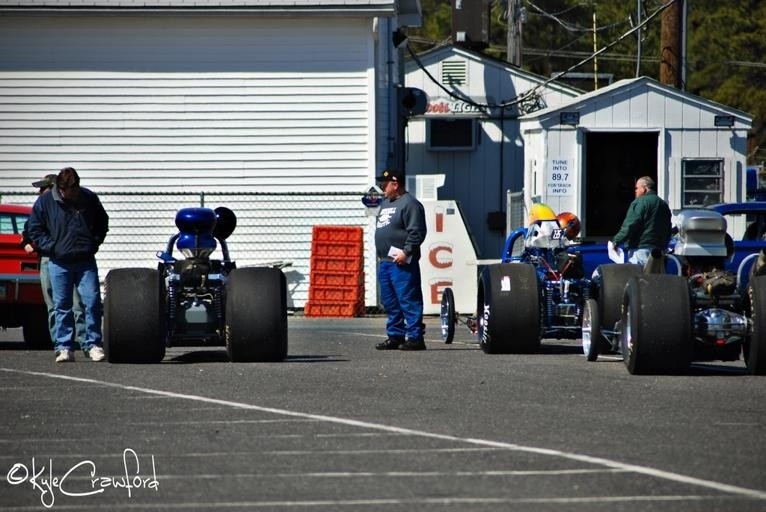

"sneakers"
<box><xmin>89</xmin><ymin>346</ymin><xmax>105</xmax><ymax>361</ymax></box>
<box><xmin>55</xmin><ymin>349</ymin><xmax>74</xmax><ymax>362</ymax></box>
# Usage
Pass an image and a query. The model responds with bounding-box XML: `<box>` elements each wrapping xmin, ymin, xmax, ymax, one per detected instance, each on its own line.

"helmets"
<box><xmin>557</xmin><ymin>212</ymin><xmax>580</xmax><ymax>240</ymax></box>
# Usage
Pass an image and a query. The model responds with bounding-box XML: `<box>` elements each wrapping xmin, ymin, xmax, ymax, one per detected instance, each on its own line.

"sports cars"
<box><xmin>438</xmin><ymin>202</ymin><xmax>644</xmax><ymax>353</ymax></box>
<box><xmin>101</xmin><ymin>204</ymin><xmax>288</xmax><ymax>363</ymax></box>
<box><xmin>580</xmin><ymin>210</ymin><xmax>766</xmax><ymax>378</ymax></box>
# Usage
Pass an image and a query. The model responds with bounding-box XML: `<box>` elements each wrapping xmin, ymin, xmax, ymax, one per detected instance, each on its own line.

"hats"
<box><xmin>376</xmin><ymin>168</ymin><xmax>405</xmax><ymax>184</ymax></box>
<box><xmin>32</xmin><ymin>175</ymin><xmax>57</xmax><ymax>187</ymax></box>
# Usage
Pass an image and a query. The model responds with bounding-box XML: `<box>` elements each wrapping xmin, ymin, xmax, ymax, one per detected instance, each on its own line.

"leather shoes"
<box><xmin>398</xmin><ymin>337</ymin><xmax>426</xmax><ymax>350</ymax></box>
<box><xmin>376</xmin><ymin>338</ymin><xmax>405</xmax><ymax>350</ymax></box>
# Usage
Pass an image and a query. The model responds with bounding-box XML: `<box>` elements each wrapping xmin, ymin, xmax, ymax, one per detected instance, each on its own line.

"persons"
<box><xmin>22</xmin><ymin>174</ymin><xmax>90</xmax><ymax>357</ymax></box>
<box><xmin>375</xmin><ymin>169</ymin><xmax>426</xmax><ymax>350</ymax></box>
<box><xmin>612</xmin><ymin>176</ymin><xmax>672</xmax><ymax>275</ymax></box>
<box><xmin>27</xmin><ymin>168</ymin><xmax>106</xmax><ymax>362</ymax></box>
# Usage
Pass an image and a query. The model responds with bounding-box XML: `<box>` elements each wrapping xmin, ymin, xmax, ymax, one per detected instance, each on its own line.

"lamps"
<box><xmin>558</xmin><ymin>112</ymin><xmax>581</xmax><ymax>131</ymax></box>
<box><xmin>711</xmin><ymin>114</ymin><xmax>739</xmax><ymax>138</ymax></box>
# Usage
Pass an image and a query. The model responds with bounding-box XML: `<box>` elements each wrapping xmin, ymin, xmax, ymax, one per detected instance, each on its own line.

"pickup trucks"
<box><xmin>1</xmin><ymin>204</ymin><xmax>49</xmax><ymax>348</ymax></box>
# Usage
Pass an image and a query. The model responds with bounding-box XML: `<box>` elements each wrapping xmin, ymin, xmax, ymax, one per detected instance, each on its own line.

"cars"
<box><xmin>567</xmin><ymin>200</ymin><xmax>766</xmax><ymax>278</ymax></box>
